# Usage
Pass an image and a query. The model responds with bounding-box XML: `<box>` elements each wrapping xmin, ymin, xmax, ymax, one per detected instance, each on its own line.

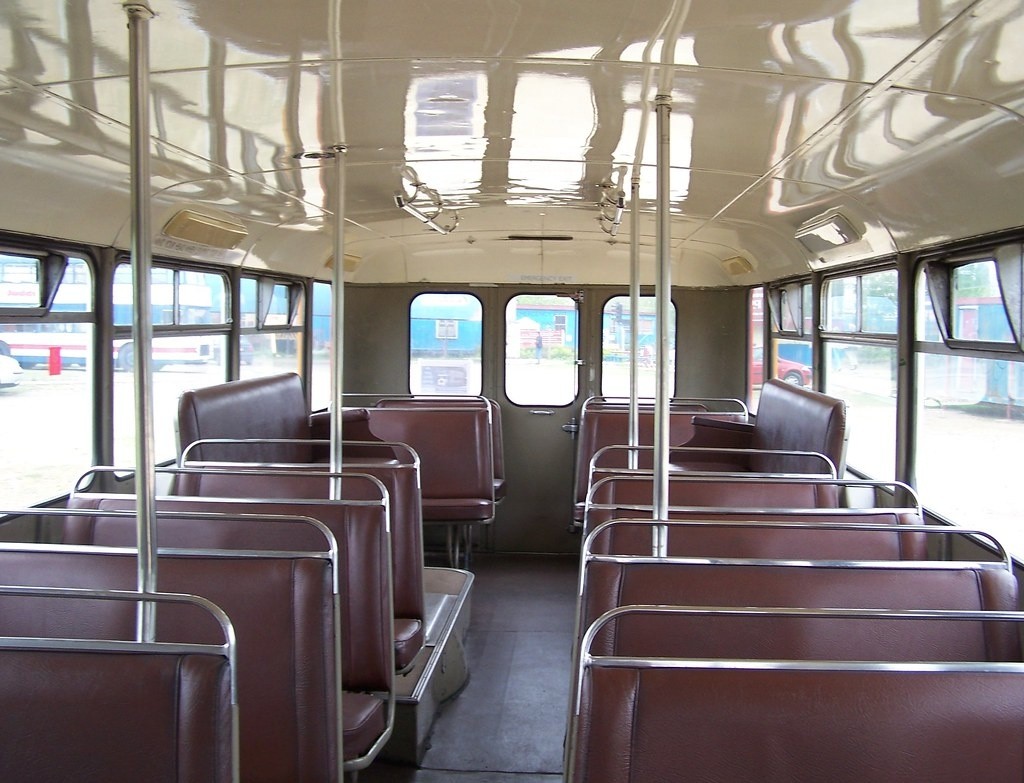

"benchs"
<box><xmin>561</xmin><ymin>379</ymin><xmax>1024</xmax><ymax>783</ymax></box>
<box><xmin>0</xmin><ymin>372</ymin><xmax>507</xmax><ymax>783</ymax></box>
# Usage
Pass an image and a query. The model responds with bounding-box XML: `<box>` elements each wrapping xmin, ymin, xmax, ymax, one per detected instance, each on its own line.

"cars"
<box><xmin>752</xmin><ymin>347</ymin><xmax>813</xmax><ymax>386</ymax></box>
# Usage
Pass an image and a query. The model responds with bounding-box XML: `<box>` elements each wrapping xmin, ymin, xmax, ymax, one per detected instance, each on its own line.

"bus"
<box><xmin>0</xmin><ymin>254</ymin><xmax>254</xmax><ymax>390</ymax></box>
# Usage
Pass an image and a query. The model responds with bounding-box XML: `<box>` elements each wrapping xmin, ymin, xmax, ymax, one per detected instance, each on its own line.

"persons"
<box><xmin>533</xmin><ymin>332</ymin><xmax>545</xmax><ymax>368</ymax></box>
